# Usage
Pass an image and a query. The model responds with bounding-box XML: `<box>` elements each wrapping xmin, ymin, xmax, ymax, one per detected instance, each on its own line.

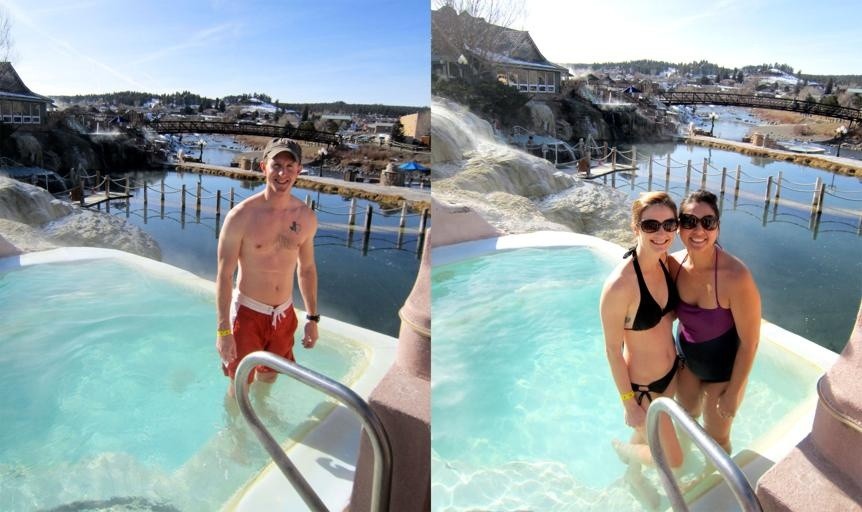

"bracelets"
<box><xmin>620</xmin><ymin>389</ymin><xmax>635</xmax><ymax>403</ymax></box>
<box><xmin>305</xmin><ymin>314</ymin><xmax>320</xmax><ymax>322</ymax></box>
<box><xmin>217</xmin><ymin>330</ymin><xmax>232</xmax><ymax>338</ymax></box>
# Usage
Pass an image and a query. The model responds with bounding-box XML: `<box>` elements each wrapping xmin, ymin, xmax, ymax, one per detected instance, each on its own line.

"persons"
<box><xmin>666</xmin><ymin>184</ymin><xmax>762</xmax><ymax>494</ymax></box>
<box><xmin>215</xmin><ymin>137</ymin><xmax>322</xmax><ymax>468</ymax></box>
<box><xmin>598</xmin><ymin>188</ymin><xmax>687</xmax><ymax>512</ymax></box>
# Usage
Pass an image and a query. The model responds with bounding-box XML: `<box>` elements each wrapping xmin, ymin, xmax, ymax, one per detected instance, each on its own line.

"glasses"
<box><xmin>637</xmin><ymin>217</ymin><xmax>680</xmax><ymax>233</ymax></box>
<box><xmin>678</xmin><ymin>213</ymin><xmax>720</xmax><ymax>232</ymax></box>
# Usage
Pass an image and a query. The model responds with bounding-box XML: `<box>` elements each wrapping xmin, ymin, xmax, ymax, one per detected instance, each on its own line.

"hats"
<box><xmin>262</xmin><ymin>136</ymin><xmax>302</xmax><ymax>166</ymax></box>
